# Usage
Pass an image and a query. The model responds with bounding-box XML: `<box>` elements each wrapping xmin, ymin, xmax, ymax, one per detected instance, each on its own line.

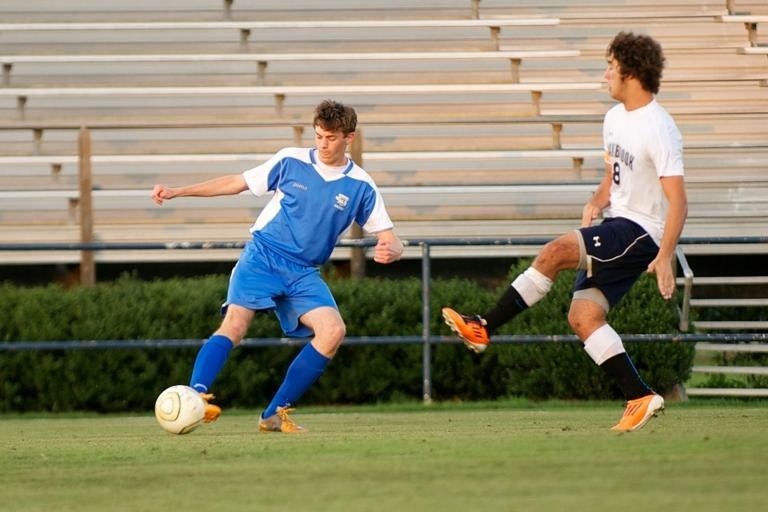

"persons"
<box><xmin>150</xmin><ymin>99</ymin><xmax>405</xmax><ymax>433</ymax></box>
<box><xmin>440</xmin><ymin>32</ymin><xmax>688</xmax><ymax>432</ymax></box>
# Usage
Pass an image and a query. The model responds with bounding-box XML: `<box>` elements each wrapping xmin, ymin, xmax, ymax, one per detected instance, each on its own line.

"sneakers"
<box><xmin>610</xmin><ymin>394</ymin><xmax>664</xmax><ymax>432</ymax></box>
<box><xmin>442</xmin><ymin>307</ymin><xmax>491</xmax><ymax>355</ymax></box>
<box><xmin>199</xmin><ymin>392</ymin><xmax>221</xmax><ymax>424</ymax></box>
<box><xmin>258</xmin><ymin>407</ymin><xmax>309</xmax><ymax>434</ymax></box>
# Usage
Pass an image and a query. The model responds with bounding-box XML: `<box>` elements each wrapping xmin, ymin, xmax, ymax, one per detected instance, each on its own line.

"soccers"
<box><xmin>155</xmin><ymin>384</ymin><xmax>206</xmax><ymax>435</ymax></box>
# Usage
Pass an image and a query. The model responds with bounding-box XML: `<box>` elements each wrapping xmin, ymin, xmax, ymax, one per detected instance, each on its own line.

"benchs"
<box><xmin>0</xmin><ymin>0</ymin><xmax>763</xmax><ymax>260</ymax></box>
